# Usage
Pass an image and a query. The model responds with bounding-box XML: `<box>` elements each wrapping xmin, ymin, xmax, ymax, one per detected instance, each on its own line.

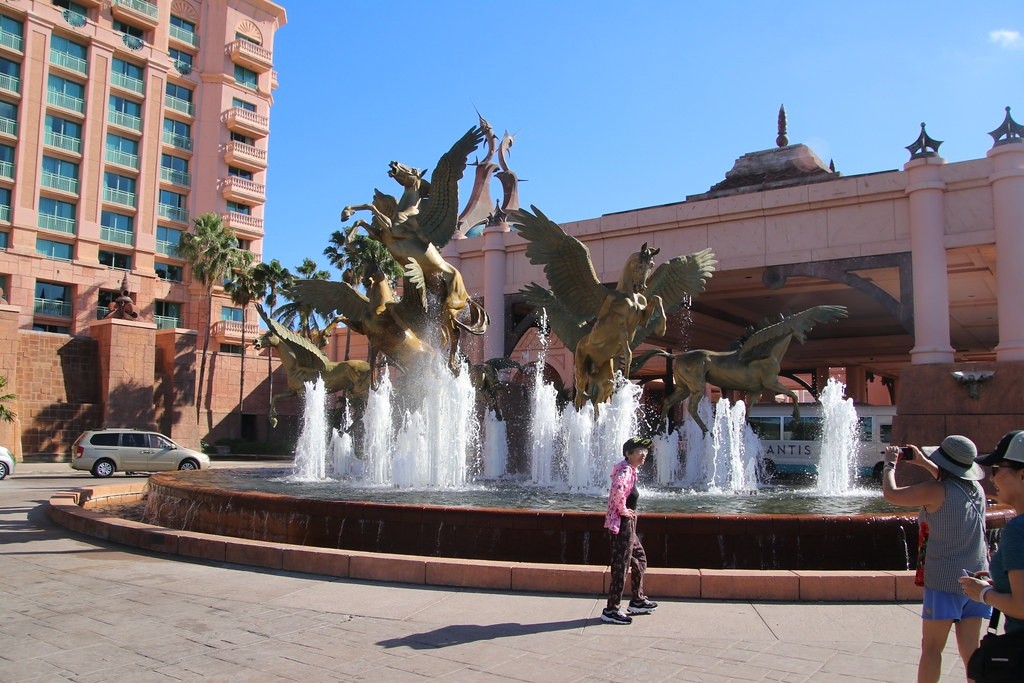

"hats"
<box><xmin>972</xmin><ymin>430</ymin><xmax>1024</xmax><ymax>469</ymax></box>
<box><xmin>623</xmin><ymin>437</ymin><xmax>653</xmax><ymax>452</ymax></box>
<box><xmin>921</xmin><ymin>434</ymin><xmax>986</xmax><ymax>481</ymax></box>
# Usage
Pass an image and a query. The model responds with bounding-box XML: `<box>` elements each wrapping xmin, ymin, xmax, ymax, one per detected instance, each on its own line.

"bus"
<box><xmin>745</xmin><ymin>401</ymin><xmax>898</xmax><ymax>486</ymax></box>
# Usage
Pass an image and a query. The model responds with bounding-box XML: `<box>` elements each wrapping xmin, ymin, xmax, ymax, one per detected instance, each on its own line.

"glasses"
<box><xmin>990</xmin><ymin>464</ymin><xmax>1024</xmax><ymax>477</ymax></box>
<box><xmin>629</xmin><ymin>449</ymin><xmax>648</xmax><ymax>454</ymax></box>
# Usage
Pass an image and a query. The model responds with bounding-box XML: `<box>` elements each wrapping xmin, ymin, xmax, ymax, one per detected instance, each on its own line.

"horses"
<box><xmin>572</xmin><ymin>242</ymin><xmax>810</xmax><ymax>439</ymax></box>
<box><xmin>251</xmin><ymin>157</ymin><xmax>506</xmax><ymax>430</ymax></box>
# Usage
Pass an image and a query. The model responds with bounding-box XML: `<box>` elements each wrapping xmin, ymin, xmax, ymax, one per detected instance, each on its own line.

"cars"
<box><xmin>0</xmin><ymin>447</ymin><xmax>18</xmax><ymax>480</ymax></box>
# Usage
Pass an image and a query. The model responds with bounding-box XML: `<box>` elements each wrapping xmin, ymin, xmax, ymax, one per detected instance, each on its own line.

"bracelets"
<box><xmin>884</xmin><ymin>468</ymin><xmax>895</xmax><ymax>474</ymax></box>
<box><xmin>979</xmin><ymin>587</ymin><xmax>994</xmax><ymax>605</ymax></box>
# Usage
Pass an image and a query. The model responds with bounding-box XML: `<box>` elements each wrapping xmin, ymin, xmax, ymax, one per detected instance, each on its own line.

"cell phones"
<box><xmin>899</xmin><ymin>447</ymin><xmax>914</xmax><ymax>460</ymax></box>
<box><xmin>962</xmin><ymin>569</ymin><xmax>981</xmax><ymax>580</ymax></box>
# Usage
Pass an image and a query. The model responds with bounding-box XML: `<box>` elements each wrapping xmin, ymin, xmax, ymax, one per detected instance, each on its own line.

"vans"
<box><xmin>70</xmin><ymin>427</ymin><xmax>211</xmax><ymax>477</ymax></box>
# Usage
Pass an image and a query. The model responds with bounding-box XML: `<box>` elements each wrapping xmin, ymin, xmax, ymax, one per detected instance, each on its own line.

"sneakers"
<box><xmin>600</xmin><ymin>608</ymin><xmax>633</xmax><ymax>625</ymax></box>
<box><xmin>626</xmin><ymin>595</ymin><xmax>659</xmax><ymax>614</ymax></box>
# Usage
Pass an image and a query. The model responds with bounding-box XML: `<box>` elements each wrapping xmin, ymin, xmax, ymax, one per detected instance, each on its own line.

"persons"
<box><xmin>883</xmin><ymin>429</ymin><xmax>1024</xmax><ymax>683</ymax></box>
<box><xmin>600</xmin><ymin>437</ymin><xmax>658</xmax><ymax>624</ymax></box>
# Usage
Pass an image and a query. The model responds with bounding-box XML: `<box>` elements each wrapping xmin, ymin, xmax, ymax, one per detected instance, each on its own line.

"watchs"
<box><xmin>884</xmin><ymin>461</ymin><xmax>896</xmax><ymax>470</ymax></box>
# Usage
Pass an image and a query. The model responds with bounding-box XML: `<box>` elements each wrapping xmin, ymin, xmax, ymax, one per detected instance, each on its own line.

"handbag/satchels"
<box><xmin>966</xmin><ymin>631</ymin><xmax>1024</xmax><ymax>683</ymax></box>
<box><xmin>987</xmin><ymin>541</ymin><xmax>999</xmax><ymax>563</ymax></box>
